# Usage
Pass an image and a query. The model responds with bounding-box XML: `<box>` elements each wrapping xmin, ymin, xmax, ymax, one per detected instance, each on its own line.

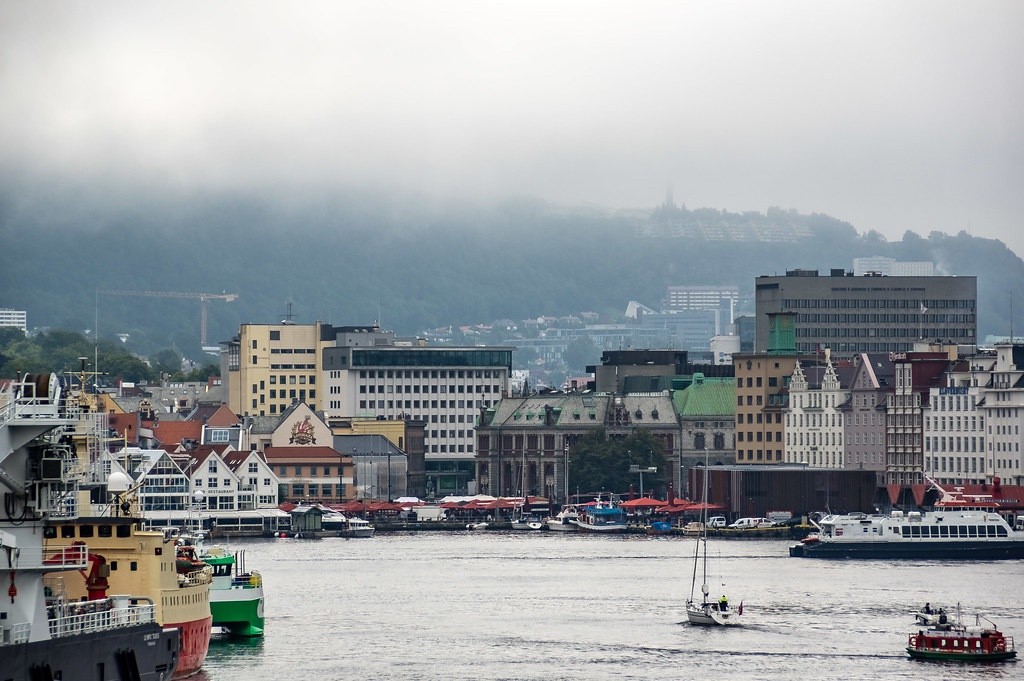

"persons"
<box><xmin>720</xmin><ymin>595</ymin><xmax>728</xmax><ymax>611</ymax></box>
<box><xmin>924</xmin><ymin>603</ymin><xmax>933</xmax><ymax>614</ymax></box>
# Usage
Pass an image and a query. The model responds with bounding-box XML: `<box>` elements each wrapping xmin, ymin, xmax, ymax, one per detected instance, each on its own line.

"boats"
<box><xmin>682</xmin><ymin>521</ymin><xmax>705</xmax><ymax>538</ymax></box>
<box><xmin>569</xmin><ymin>492</ymin><xmax>628</xmax><ymax>534</ymax></box>
<box><xmin>906</xmin><ymin>602</ymin><xmax>1018</xmax><ymax>663</ymax></box>
<box><xmin>1</xmin><ymin>374</ymin><xmax>267</xmax><ymax>681</ymax></box>
<box><xmin>547</xmin><ymin>506</ymin><xmax>580</xmax><ymax>532</ymax></box>
<box><xmin>788</xmin><ymin>470</ymin><xmax>1024</xmax><ymax>560</ymax></box>
<box><xmin>465</xmin><ymin>521</ymin><xmax>490</xmax><ymax>531</ymax></box>
<box><xmin>337</xmin><ymin>515</ymin><xmax>375</xmax><ymax>537</ymax></box>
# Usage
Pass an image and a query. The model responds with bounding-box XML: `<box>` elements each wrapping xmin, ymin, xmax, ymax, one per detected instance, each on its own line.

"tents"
<box><xmin>279</xmin><ymin>501</ymin><xmax>424</xmax><ymax>526</ymax></box>
<box><xmin>394</xmin><ymin>495</ymin><xmax>550</xmax><ymax>523</ymax></box>
<box><xmin>562</xmin><ymin>498</ymin><xmax>723</xmax><ymax>526</ymax></box>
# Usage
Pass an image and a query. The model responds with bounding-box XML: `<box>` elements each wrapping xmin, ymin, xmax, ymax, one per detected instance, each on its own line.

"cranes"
<box><xmin>96</xmin><ymin>287</ymin><xmax>238</xmax><ymax>344</ymax></box>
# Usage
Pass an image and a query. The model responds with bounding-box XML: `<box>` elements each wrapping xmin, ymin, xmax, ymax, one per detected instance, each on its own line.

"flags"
<box><xmin>739</xmin><ymin>602</ymin><xmax>742</xmax><ymax>615</ymax></box>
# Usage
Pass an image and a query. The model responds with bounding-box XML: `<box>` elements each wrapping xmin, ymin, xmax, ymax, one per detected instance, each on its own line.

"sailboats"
<box><xmin>683</xmin><ymin>444</ymin><xmax>753</xmax><ymax>627</ymax></box>
<box><xmin>510</xmin><ymin>429</ymin><xmax>542</xmax><ymax>530</ymax></box>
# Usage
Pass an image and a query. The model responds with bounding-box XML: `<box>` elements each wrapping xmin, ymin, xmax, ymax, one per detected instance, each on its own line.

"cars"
<box><xmin>706</xmin><ymin>515</ymin><xmax>801</xmax><ymax>528</ymax></box>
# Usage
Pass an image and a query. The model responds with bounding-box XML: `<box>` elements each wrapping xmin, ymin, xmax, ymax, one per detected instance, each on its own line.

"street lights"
<box><xmin>680</xmin><ymin>465</ymin><xmax>684</xmax><ymax>499</ymax></box>
<box><xmin>386</xmin><ymin>451</ymin><xmax>392</xmax><ymax>501</ymax></box>
